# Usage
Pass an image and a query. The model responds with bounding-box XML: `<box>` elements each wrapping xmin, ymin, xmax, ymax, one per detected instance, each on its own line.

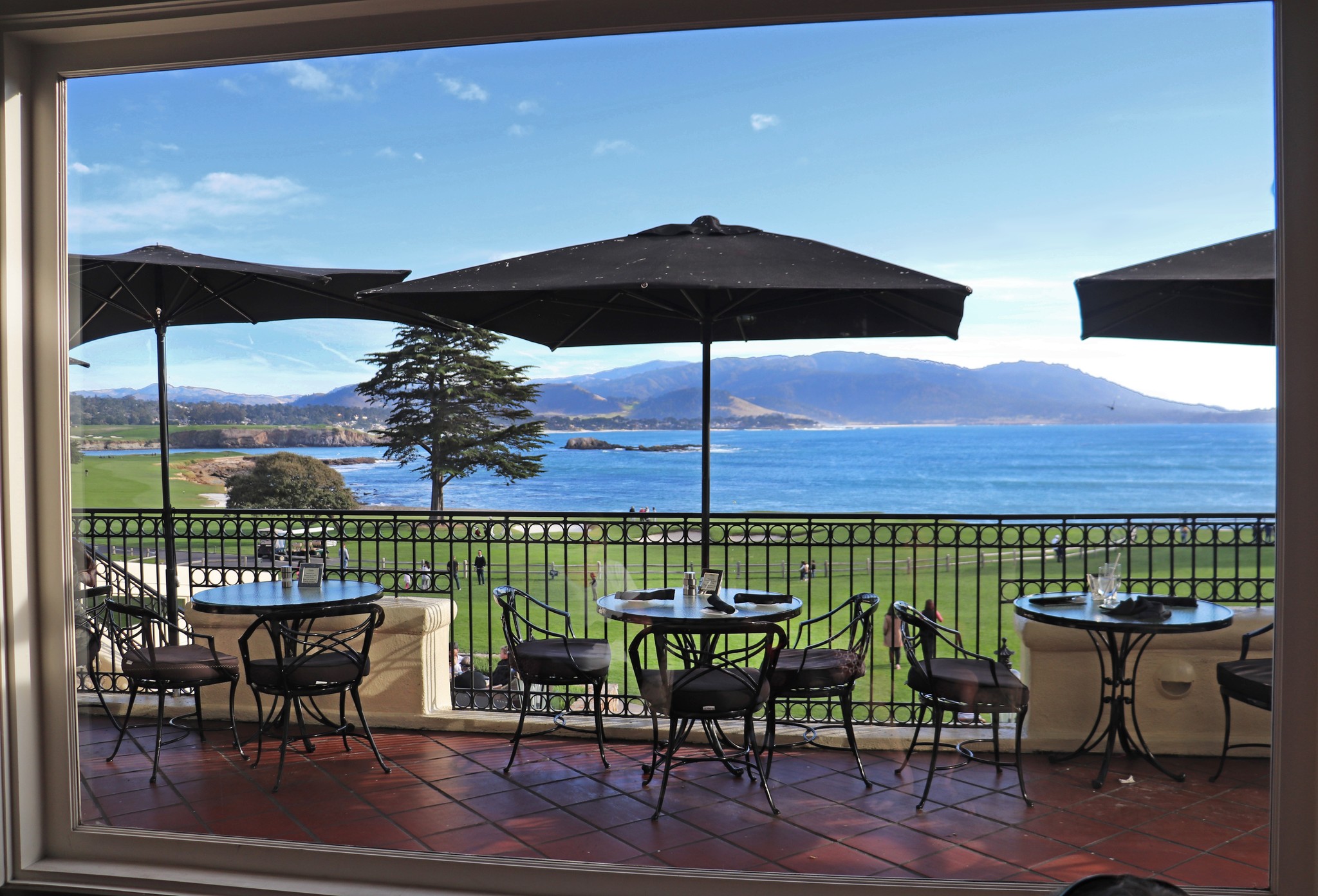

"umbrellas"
<box><xmin>351</xmin><ymin>215</ymin><xmax>972</xmax><ymax>669</ymax></box>
<box><xmin>68</xmin><ymin>242</ymin><xmax>462</xmax><ymax>702</ymax></box>
<box><xmin>1074</xmin><ymin>229</ymin><xmax>1275</xmax><ymax>346</ymax></box>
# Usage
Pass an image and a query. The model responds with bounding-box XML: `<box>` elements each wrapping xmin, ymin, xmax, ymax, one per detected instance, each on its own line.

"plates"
<box><xmin>700</xmin><ymin>608</ymin><xmax>739</xmax><ymax>615</ymax></box>
<box><xmin>622</xmin><ymin>599</ymin><xmax>653</xmax><ymax>603</ymax></box>
<box><xmin>749</xmin><ymin>602</ymin><xmax>780</xmax><ymax>606</ymax></box>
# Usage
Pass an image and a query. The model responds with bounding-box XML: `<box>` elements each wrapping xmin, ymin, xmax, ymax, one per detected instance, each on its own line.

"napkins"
<box><xmin>703</xmin><ymin>593</ymin><xmax>735</xmax><ymax>614</ymax></box>
<box><xmin>1137</xmin><ymin>595</ymin><xmax>1198</xmax><ymax>607</ymax></box>
<box><xmin>733</xmin><ymin>593</ymin><xmax>793</xmax><ymax>604</ymax></box>
<box><xmin>614</xmin><ymin>589</ymin><xmax>675</xmax><ymax>600</ymax></box>
<box><xmin>1101</xmin><ymin>598</ymin><xmax>1172</xmax><ymax>621</ymax></box>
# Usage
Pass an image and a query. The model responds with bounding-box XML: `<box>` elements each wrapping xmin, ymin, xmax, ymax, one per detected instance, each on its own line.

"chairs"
<box><xmin>451</xmin><ymin>585</ymin><xmax>879</xmax><ymax>822</ymax></box>
<box><xmin>286</xmin><ymin>540</ymin><xmax>321</xmax><ymax>552</ymax></box>
<box><xmin>894</xmin><ymin>601</ymin><xmax>1034</xmax><ymax>810</ymax></box>
<box><xmin>74</xmin><ymin>585</ymin><xmax>393</xmax><ymax>793</ymax></box>
<box><xmin>1209</xmin><ymin>623</ymin><xmax>1274</xmax><ymax>780</ymax></box>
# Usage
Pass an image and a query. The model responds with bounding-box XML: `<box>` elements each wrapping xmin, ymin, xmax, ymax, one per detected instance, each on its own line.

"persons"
<box><xmin>404</xmin><ymin>569</ymin><xmax>413</xmax><ymax>589</ymax></box>
<box><xmin>589</xmin><ymin>572</ymin><xmax>597</xmax><ymax>601</ymax></box>
<box><xmin>447</xmin><ymin>555</ymin><xmax>461</xmax><ymax>590</ymax></box>
<box><xmin>798</xmin><ymin>560</ymin><xmax>816</xmax><ymax>581</ymax></box>
<box><xmin>629</xmin><ymin>505</ymin><xmax>656</xmax><ymax>521</ymax></box>
<box><xmin>883</xmin><ymin>602</ymin><xmax>904</xmax><ymax>669</ymax></box>
<box><xmin>486</xmin><ymin>645</ymin><xmax>517</xmax><ymax>689</ymax></box>
<box><xmin>474</xmin><ymin>550</ymin><xmax>486</xmax><ymax>585</ymax></box>
<box><xmin>338</xmin><ymin>543</ymin><xmax>349</xmax><ymax>575</ymax></box>
<box><xmin>419</xmin><ymin>560</ymin><xmax>431</xmax><ymax>591</ymax></box>
<box><xmin>530</xmin><ymin>683</ymin><xmax>553</xmax><ymax>709</ymax></box>
<box><xmin>72</xmin><ymin>537</ymin><xmax>102</xmax><ymax>667</ymax></box>
<box><xmin>449</xmin><ymin>641</ymin><xmax>464</xmax><ymax>674</ymax></box>
<box><xmin>919</xmin><ymin>599</ymin><xmax>943</xmax><ymax>658</ymax></box>
<box><xmin>450</xmin><ymin>656</ymin><xmax>487</xmax><ymax>689</ymax></box>
<box><xmin>296</xmin><ymin>559</ymin><xmax>305</xmax><ymax>579</ymax></box>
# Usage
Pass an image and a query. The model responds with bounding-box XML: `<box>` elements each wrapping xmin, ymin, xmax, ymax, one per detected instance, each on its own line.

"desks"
<box><xmin>597</xmin><ymin>588</ymin><xmax>802</xmax><ymax>773</ymax></box>
<box><xmin>191</xmin><ymin>579</ymin><xmax>393</xmax><ymax>794</ymax></box>
<box><xmin>1013</xmin><ymin>591</ymin><xmax>1234</xmax><ymax>789</ymax></box>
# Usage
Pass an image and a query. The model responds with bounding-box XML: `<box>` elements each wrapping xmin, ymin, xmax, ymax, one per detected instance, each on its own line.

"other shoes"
<box><xmin>896</xmin><ymin>664</ymin><xmax>900</xmax><ymax>670</ymax></box>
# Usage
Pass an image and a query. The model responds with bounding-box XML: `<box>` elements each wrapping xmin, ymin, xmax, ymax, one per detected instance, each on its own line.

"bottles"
<box><xmin>683</xmin><ymin>571</ymin><xmax>697</xmax><ymax>595</ymax></box>
<box><xmin>281</xmin><ymin>565</ymin><xmax>292</xmax><ymax>587</ymax></box>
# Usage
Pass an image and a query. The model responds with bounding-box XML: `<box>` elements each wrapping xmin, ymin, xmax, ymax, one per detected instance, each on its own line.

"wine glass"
<box><xmin>1105</xmin><ymin>561</ymin><xmax>1122</xmax><ymax>604</ymax></box>
<box><xmin>1098</xmin><ymin>566</ymin><xmax>1116</xmax><ymax>609</ymax></box>
